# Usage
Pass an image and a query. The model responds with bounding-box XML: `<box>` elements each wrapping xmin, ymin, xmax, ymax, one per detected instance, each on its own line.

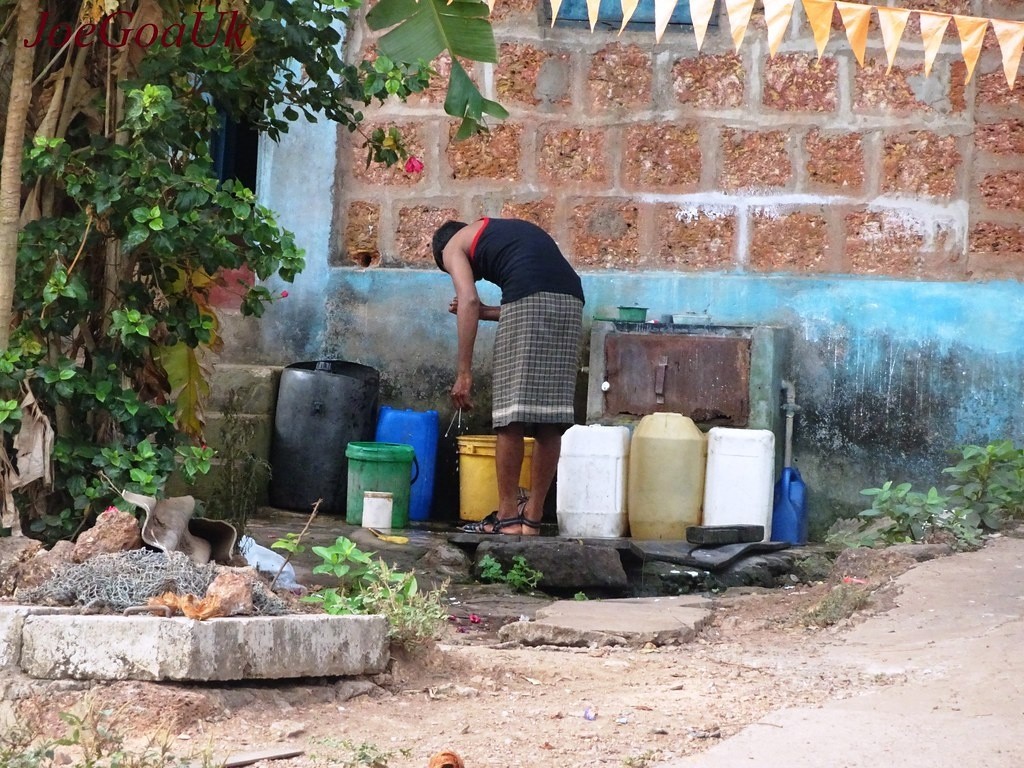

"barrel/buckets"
<box><xmin>376</xmin><ymin>405</ymin><xmax>440</xmax><ymax>521</ymax></box>
<box><xmin>773</xmin><ymin>467</ymin><xmax>808</xmax><ymax>547</ymax></box>
<box><xmin>555</xmin><ymin>423</ymin><xmax>630</xmax><ymax>537</ymax></box>
<box><xmin>627</xmin><ymin>411</ymin><xmax>703</xmax><ymax>542</ymax></box>
<box><xmin>455</xmin><ymin>434</ymin><xmax>535</xmax><ymax>521</ymax></box>
<box><xmin>703</xmin><ymin>427</ymin><xmax>776</xmax><ymax>542</ymax></box>
<box><xmin>271</xmin><ymin>361</ymin><xmax>375</xmax><ymax>511</ymax></box>
<box><xmin>344</xmin><ymin>441</ymin><xmax>420</xmax><ymax>530</ymax></box>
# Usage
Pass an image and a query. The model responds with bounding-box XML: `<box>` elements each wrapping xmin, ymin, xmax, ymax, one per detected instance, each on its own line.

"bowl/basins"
<box><xmin>672</xmin><ymin>314</ymin><xmax>708</xmax><ymax>325</ymax></box>
<box><xmin>617</xmin><ymin>305</ymin><xmax>649</xmax><ymax>322</ymax></box>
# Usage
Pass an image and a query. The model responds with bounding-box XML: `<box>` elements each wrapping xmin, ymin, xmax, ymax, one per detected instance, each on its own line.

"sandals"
<box><xmin>454</xmin><ymin>495</ymin><xmax>542</xmax><ymax>536</ymax></box>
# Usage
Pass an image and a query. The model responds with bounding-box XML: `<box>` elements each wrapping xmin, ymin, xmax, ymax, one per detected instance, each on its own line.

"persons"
<box><xmin>432</xmin><ymin>217</ymin><xmax>586</xmax><ymax>535</ymax></box>
